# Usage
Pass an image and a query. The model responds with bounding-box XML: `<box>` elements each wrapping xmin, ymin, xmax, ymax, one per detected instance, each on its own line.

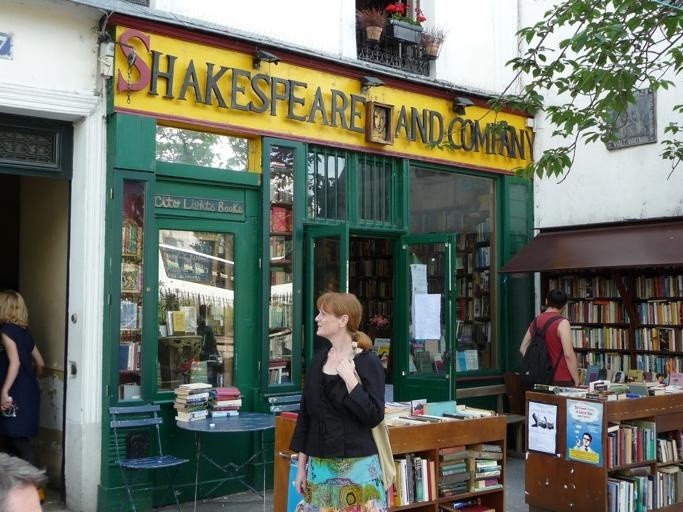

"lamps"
<box><xmin>453</xmin><ymin>95</ymin><xmax>474</xmax><ymax>115</ymax></box>
<box><xmin>252</xmin><ymin>50</ymin><xmax>281</xmax><ymax>69</ymax></box>
<box><xmin>361</xmin><ymin>75</ymin><xmax>385</xmax><ymax>89</ymax></box>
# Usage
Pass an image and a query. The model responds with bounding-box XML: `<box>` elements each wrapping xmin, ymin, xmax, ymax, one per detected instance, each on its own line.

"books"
<box><xmin>270</xmin><ymin>236</ymin><xmax>292</xmax><ymax>261</ymax></box>
<box><xmin>270</xmin><ymin>205</ymin><xmax>294</xmax><ymax>234</ymax></box>
<box><xmin>549</xmin><ymin>274</ymin><xmax>682</xmax><ymax>399</ymax></box>
<box><xmin>120</xmin><ymin>225</ymin><xmax>144</xmax><ymax>373</ymax></box>
<box><xmin>159</xmin><ymin>305</ymin><xmax>198</xmax><ymax>338</ymax></box>
<box><xmin>349</xmin><ymin>238</ymin><xmax>393</xmax><ymax>368</ymax></box>
<box><xmin>210</xmin><ymin>387</ymin><xmax>243</xmax><ymax>418</ymax></box>
<box><xmin>408</xmin><ymin>396</ymin><xmax>458</xmax><ymax>417</ymax></box>
<box><xmin>394</xmin><ymin>444</ymin><xmax>503</xmax><ymax>512</ymax></box>
<box><xmin>456</xmin><ymin>217</ymin><xmax>490</xmax><ymax>369</ymax></box>
<box><xmin>173</xmin><ymin>383</ymin><xmax>212</xmax><ymax>424</ymax></box>
<box><xmin>190</xmin><ymin>360</ymin><xmax>219</xmax><ymax>386</ymax></box>
<box><xmin>269</xmin><ymin>366</ymin><xmax>287</xmax><ymax>386</ymax></box>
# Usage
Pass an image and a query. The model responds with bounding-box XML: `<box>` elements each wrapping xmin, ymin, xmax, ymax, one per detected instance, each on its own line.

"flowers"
<box><xmin>385</xmin><ymin>2</ymin><xmax>426</xmax><ymax>25</ymax></box>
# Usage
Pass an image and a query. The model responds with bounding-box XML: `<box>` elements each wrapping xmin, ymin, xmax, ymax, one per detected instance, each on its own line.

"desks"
<box><xmin>177</xmin><ymin>411</ymin><xmax>278</xmax><ymax>512</ymax></box>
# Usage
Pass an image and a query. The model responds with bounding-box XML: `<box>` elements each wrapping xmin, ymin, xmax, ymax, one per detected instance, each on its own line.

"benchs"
<box><xmin>456</xmin><ymin>383</ymin><xmax>523</xmax><ymax>456</ymax></box>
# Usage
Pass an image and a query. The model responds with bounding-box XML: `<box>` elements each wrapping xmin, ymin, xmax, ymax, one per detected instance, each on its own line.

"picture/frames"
<box><xmin>366</xmin><ymin>100</ymin><xmax>394</xmax><ymax>145</ymax></box>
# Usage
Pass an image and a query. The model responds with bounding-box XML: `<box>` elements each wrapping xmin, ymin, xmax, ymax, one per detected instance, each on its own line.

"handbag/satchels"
<box><xmin>371</xmin><ymin>421</ymin><xmax>397</xmax><ymax>491</ymax></box>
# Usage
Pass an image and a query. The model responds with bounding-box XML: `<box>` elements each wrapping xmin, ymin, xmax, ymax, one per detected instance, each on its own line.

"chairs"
<box><xmin>269</xmin><ymin>394</ymin><xmax>301</xmax><ymax>413</ymax></box>
<box><xmin>108</xmin><ymin>404</ymin><xmax>189</xmax><ymax>512</ymax></box>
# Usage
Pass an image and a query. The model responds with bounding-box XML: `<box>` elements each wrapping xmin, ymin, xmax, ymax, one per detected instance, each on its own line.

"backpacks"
<box><xmin>520</xmin><ymin>315</ymin><xmax>563</xmax><ymax>386</ymax></box>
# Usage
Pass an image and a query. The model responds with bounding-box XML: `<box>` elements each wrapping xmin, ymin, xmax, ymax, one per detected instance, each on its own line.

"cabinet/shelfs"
<box><xmin>118</xmin><ymin>179</ymin><xmax>305</xmax><ymax>400</ymax></box>
<box><xmin>537</xmin><ymin>264</ymin><xmax>683</xmax><ymax>383</ymax></box>
<box><xmin>272</xmin><ymin>407</ymin><xmax>506</xmax><ymax>512</ymax></box>
<box><xmin>523</xmin><ymin>390</ymin><xmax>683</xmax><ymax>512</ymax></box>
<box><xmin>314</xmin><ymin>174</ymin><xmax>496</xmax><ymax>370</ymax></box>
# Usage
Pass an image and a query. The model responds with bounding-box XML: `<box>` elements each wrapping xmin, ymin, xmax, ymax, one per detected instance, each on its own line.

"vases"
<box><xmin>387</xmin><ymin>18</ymin><xmax>423</xmax><ymax>44</ymax></box>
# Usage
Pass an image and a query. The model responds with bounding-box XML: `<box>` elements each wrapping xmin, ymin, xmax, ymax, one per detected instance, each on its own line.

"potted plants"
<box><xmin>420</xmin><ymin>25</ymin><xmax>447</xmax><ymax>60</ymax></box>
<box><xmin>356</xmin><ymin>5</ymin><xmax>387</xmax><ymax>42</ymax></box>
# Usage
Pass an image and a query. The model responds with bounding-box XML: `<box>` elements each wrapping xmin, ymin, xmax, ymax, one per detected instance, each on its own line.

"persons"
<box><xmin>519</xmin><ymin>288</ymin><xmax>579</xmax><ymax>387</ymax></box>
<box><xmin>372</xmin><ymin>105</ymin><xmax>389</xmax><ymax>141</ymax></box>
<box><xmin>288</xmin><ymin>293</ymin><xmax>387</xmax><ymax>512</ymax></box>
<box><xmin>573</xmin><ymin>433</ymin><xmax>596</xmax><ymax>453</ymax></box>
<box><xmin>0</xmin><ymin>452</ymin><xmax>49</xmax><ymax>512</ymax></box>
<box><xmin>607</xmin><ymin>420</ymin><xmax>683</xmax><ymax>512</ymax></box>
<box><xmin>0</xmin><ymin>290</ymin><xmax>45</xmax><ymax>468</ymax></box>
